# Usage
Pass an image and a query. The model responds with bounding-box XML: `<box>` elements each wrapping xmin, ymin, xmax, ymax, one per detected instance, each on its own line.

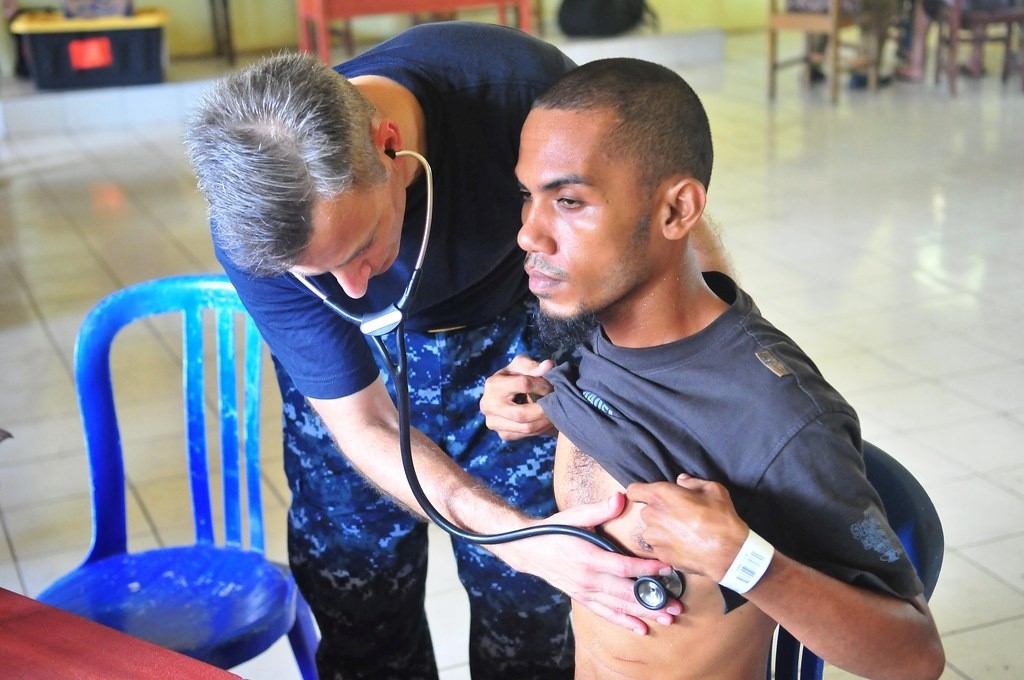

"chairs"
<box><xmin>35</xmin><ymin>274</ymin><xmax>318</xmax><ymax>680</ymax></box>
<box><xmin>767</xmin><ymin>0</ymin><xmax>1024</xmax><ymax>105</ymax></box>
<box><xmin>766</xmin><ymin>440</ymin><xmax>944</xmax><ymax>680</ymax></box>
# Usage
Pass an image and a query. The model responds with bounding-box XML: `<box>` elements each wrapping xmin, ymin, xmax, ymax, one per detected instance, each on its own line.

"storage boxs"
<box><xmin>10</xmin><ymin>7</ymin><xmax>167</xmax><ymax>90</ymax></box>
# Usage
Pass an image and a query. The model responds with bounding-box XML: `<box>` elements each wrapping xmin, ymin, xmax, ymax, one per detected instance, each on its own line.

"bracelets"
<box><xmin>721</xmin><ymin>530</ymin><xmax>776</xmax><ymax>597</ymax></box>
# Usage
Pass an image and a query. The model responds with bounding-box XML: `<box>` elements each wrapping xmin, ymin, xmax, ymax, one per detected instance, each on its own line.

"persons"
<box><xmin>480</xmin><ymin>57</ymin><xmax>946</xmax><ymax>679</ymax></box>
<box><xmin>784</xmin><ymin>0</ymin><xmax>1024</xmax><ymax>89</ymax></box>
<box><xmin>186</xmin><ymin>20</ymin><xmax>734</xmax><ymax>680</ymax></box>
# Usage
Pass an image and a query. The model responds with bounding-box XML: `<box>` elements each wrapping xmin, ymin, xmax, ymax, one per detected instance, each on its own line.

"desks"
<box><xmin>0</xmin><ymin>587</ymin><xmax>244</xmax><ymax>680</ymax></box>
<box><xmin>297</xmin><ymin>0</ymin><xmax>531</xmax><ymax>64</ymax></box>
